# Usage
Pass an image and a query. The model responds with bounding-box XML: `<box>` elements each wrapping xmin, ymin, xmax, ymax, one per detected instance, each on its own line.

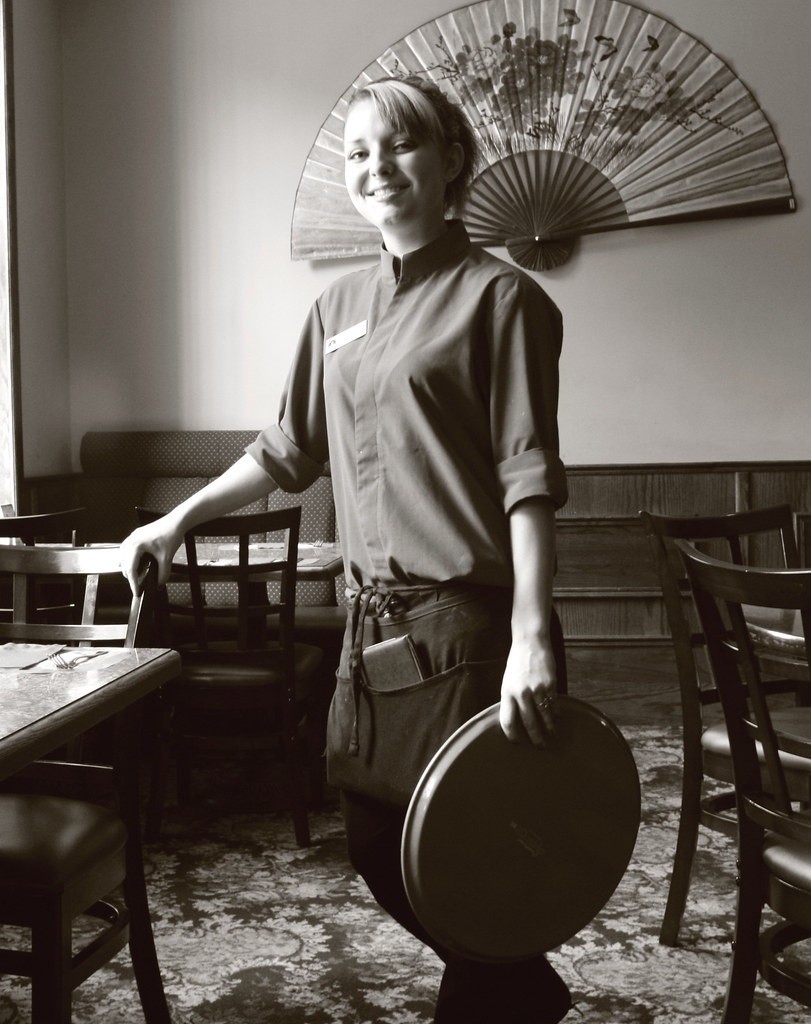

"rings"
<box><xmin>537</xmin><ymin>696</ymin><xmax>555</xmax><ymax>712</ymax></box>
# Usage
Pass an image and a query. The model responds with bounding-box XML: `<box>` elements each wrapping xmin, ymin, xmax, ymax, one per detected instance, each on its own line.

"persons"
<box><xmin>117</xmin><ymin>73</ymin><xmax>579</xmax><ymax>1024</ymax></box>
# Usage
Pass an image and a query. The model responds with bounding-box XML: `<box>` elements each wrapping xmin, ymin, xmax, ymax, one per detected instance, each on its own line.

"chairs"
<box><xmin>0</xmin><ymin>507</ymin><xmax>327</xmax><ymax>1024</ymax></box>
<box><xmin>637</xmin><ymin>505</ymin><xmax>811</xmax><ymax>1024</ymax></box>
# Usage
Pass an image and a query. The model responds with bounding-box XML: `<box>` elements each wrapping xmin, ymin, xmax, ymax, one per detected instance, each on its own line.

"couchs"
<box><xmin>16</xmin><ymin>431</ymin><xmax>351</xmax><ymax>643</ymax></box>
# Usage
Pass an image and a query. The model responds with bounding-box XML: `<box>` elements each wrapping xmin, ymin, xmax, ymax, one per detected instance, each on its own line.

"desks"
<box><xmin>0</xmin><ymin>643</ymin><xmax>365</xmax><ymax>1024</ymax></box>
<box><xmin>728</xmin><ymin>594</ymin><xmax>811</xmax><ymax>679</ymax></box>
<box><xmin>0</xmin><ymin>543</ymin><xmax>345</xmax><ymax>817</ymax></box>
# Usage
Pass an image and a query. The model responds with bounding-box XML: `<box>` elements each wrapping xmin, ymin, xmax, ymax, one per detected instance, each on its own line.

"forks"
<box><xmin>309</xmin><ymin>538</ymin><xmax>323</xmax><ymax>548</ymax></box>
<box><xmin>48</xmin><ymin>650</ymin><xmax>109</xmax><ymax>668</ymax></box>
